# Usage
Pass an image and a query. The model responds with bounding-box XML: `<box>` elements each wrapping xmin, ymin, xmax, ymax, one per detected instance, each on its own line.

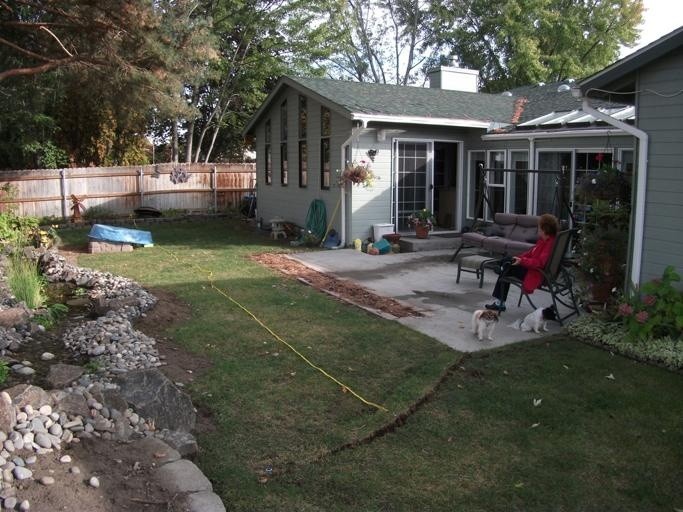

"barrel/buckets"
<box><xmin>384</xmin><ymin>234</ymin><xmax>401</xmax><ymax>244</ymax></box>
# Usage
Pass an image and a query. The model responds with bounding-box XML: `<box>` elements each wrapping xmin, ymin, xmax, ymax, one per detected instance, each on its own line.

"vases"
<box><xmin>590</xmin><ymin>278</ymin><xmax>612</xmax><ymax>301</ymax></box>
<box><xmin>415</xmin><ymin>225</ymin><xmax>429</xmax><ymax>239</ymax></box>
<box><xmin>591</xmin><ymin>186</ymin><xmax>620</xmax><ymax>199</ymax></box>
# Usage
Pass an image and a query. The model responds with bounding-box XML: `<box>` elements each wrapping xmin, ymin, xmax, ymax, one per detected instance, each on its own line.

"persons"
<box><xmin>484</xmin><ymin>213</ymin><xmax>559</xmax><ymax>312</ymax></box>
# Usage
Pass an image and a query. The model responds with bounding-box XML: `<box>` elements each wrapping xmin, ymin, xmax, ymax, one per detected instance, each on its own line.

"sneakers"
<box><xmin>485</xmin><ymin>300</ymin><xmax>507</xmax><ymax>312</ymax></box>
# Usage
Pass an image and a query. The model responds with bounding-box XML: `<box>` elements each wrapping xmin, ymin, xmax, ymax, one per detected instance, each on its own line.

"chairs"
<box><xmin>498</xmin><ymin>230</ymin><xmax>580</xmax><ymax>327</ymax></box>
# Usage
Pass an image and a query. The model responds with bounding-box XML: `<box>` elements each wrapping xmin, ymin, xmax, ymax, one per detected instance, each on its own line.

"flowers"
<box><xmin>577</xmin><ymin>166</ymin><xmax>630</xmax><ymax>196</ymax></box>
<box><xmin>408</xmin><ymin>208</ymin><xmax>436</xmax><ymax>231</ymax></box>
<box><xmin>335</xmin><ymin>159</ymin><xmax>381</xmax><ymax>190</ymax></box>
<box><xmin>572</xmin><ymin>228</ymin><xmax>626</xmax><ymax>282</ymax></box>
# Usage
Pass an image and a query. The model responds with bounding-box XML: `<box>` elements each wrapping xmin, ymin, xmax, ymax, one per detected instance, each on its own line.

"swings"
<box><xmin>461</xmin><ymin>171</ymin><xmax>565</xmax><ymax>259</ymax></box>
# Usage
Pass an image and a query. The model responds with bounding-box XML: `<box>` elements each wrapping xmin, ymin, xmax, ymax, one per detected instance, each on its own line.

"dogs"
<box><xmin>470</xmin><ymin>308</ymin><xmax>499</xmax><ymax>342</ymax></box>
<box><xmin>508</xmin><ymin>303</ymin><xmax>559</xmax><ymax>334</ymax></box>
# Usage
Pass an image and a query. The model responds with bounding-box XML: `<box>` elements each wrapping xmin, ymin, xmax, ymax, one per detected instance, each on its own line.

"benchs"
<box><xmin>462</xmin><ymin>213</ymin><xmax>568</xmax><ymax>256</ymax></box>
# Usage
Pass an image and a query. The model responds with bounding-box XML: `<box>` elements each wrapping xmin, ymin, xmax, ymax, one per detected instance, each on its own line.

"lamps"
<box><xmin>368</xmin><ymin>146</ymin><xmax>377</xmax><ymax>161</ymax></box>
<box><xmin>571</xmin><ymin>86</ymin><xmax>584</xmax><ymax>102</ymax></box>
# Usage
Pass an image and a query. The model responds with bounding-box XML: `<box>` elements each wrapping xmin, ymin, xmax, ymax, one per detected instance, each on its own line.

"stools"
<box><xmin>457</xmin><ymin>255</ymin><xmax>502</xmax><ymax>288</ymax></box>
